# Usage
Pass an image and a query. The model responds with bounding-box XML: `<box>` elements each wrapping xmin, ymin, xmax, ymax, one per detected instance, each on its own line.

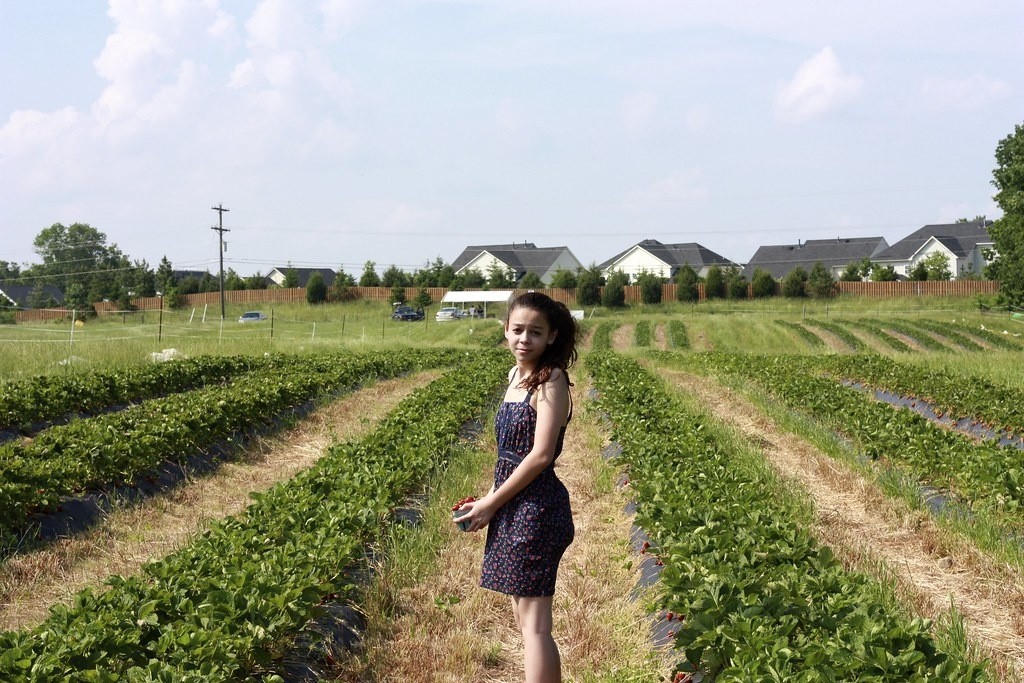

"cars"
<box><xmin>391</xmin><ymin>306</ymin><xmax>426</xmax><ymax>322</ymax></box>
<box><xmin>436</xmin><ymin>306</ymin><xmax>468</xmax><ymax>321</ymax></box>
<box><xmin>238</xmin><ymin>311</ymin><xmax>267</xmax><ymax>323</ymax></box>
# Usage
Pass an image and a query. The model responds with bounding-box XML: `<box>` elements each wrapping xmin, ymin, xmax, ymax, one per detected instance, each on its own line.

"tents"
<box><xmin>440</xmin><ymin>291</ymin><xmax>514</xmax><ymax>318</ymax></box>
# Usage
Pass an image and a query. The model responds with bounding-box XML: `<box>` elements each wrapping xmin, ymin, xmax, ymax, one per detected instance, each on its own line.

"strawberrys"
<box><xmin>452</xmin><ymin>495</ymin><xmax>475</xmax><ymax>511</ymax></box>
<box><xmin>640</xmin><ymin>541</ymin><xmax>692</xmax><ymax>683</ymax></box>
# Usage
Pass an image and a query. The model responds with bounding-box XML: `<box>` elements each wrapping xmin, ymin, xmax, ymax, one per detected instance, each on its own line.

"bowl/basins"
<box><xmin>452</xmin><ymin>500</ymin><xmax>477</xmax><ymax>532</ymax></box>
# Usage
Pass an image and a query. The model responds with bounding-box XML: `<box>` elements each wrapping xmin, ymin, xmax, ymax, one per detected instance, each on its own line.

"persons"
<box><xmin>469</xmin><ymin>305</ymin><xmax>476</xmax><ymax>321</ymax></box>
<box><xmin>477</xmin><ymin>305</ymin><xmax>484</xmax><ymax>318</ymax></box>
<box><xmin>451</xmin><ymin>292</ymin><xmax>582</xmax><ymax>683</ymax></box>
